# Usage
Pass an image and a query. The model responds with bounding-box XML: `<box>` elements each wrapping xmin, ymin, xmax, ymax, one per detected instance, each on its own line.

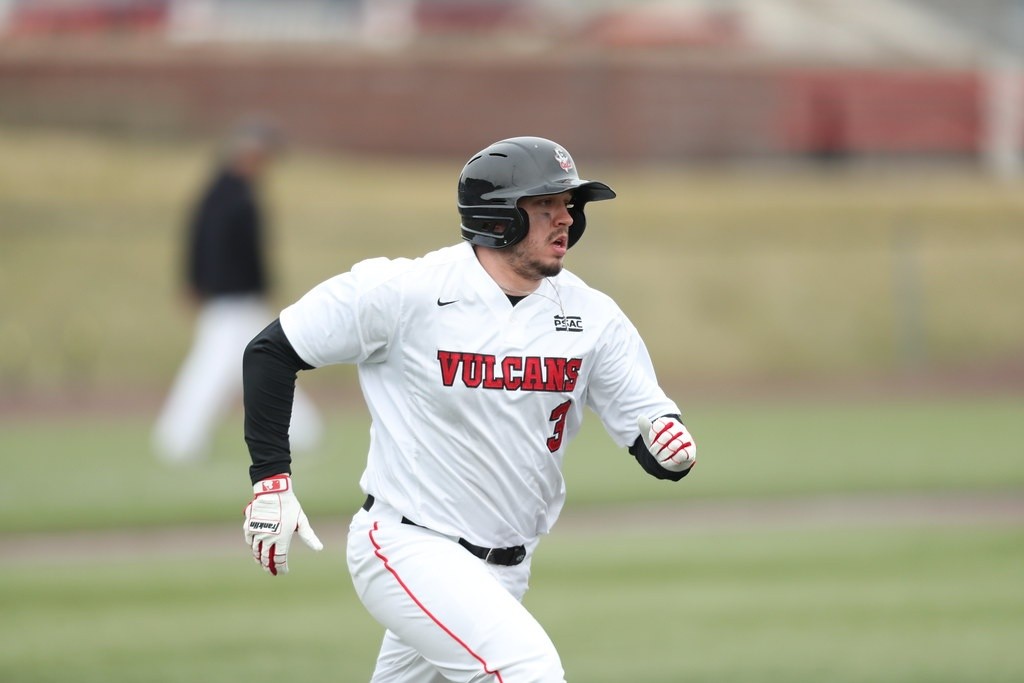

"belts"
<box><xmin>457</xmin><ymin>536</ymin><xmax>526</xmax><ymax>567</ymax></box>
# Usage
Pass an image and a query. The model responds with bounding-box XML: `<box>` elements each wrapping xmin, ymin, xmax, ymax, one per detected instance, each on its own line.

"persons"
<box><xmin>151</xmin><ymin>134</ymin><xmax>323</xmax><ymax>460</ymax></box>
<box><xmin>240</xmin><ymin>135</ymin><xmax>698</xmax><ymax>681</ymax></box>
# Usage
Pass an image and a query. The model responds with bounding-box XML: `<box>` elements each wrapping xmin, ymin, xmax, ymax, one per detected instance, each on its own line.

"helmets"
<box><xmin>457</xmin><ymin>136</ymin><xmax>617</xmax><ymax>250</ymax></box>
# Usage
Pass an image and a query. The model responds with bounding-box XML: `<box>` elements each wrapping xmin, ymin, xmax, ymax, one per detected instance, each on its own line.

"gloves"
<box><xmin>243</xmin><ymin>473</ymin><xmax>324</xmax><ymax>576</ymax></box>
<box><xmin>636</xmin><ymin>414</ymin><xmax>697</xmax><ymax>473</ymax></box>
